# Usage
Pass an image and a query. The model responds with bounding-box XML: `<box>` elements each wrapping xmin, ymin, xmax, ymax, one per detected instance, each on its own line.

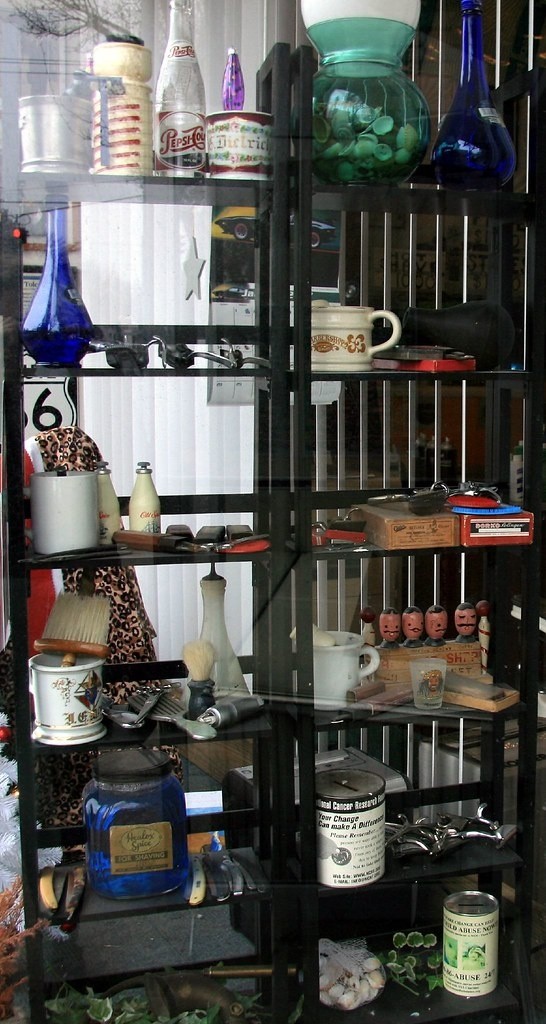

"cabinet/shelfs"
<box><xmin>0</xmin><ymin>44</ymin><xmax>546</xmax><ymax>1024</ymax></box>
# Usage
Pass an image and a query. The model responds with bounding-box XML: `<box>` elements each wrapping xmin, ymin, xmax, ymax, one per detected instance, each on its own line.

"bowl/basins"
<box><xmin>205</xmin><ymin>111</ymin><xmax>272</xmax><ymax>180</ymax></box>
<box><xmin>18</xmin><ymin>95</ymin><xmax>91</xmax><ymax>175</ymax></box>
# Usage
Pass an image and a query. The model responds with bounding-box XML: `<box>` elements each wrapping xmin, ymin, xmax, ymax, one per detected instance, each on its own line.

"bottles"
<box><xmin>222</xmin><ymin>48</ymin><xmax>245</xmax><ymax>111</ymax></box>
<box><xmin>183</xmin><ymin>562</ymin><xmax>249</xmax><ymax>704</ymax></box>
<box><xmin>91</xmin><ymin>461</ymin><xmax>120</xmax><ymax>545</ymax></box>
<box><xmin>19</xmin><ymin>201</ymin><xmax>94</xmax><ymax>369</ymax></box>
<box><xmin>431</xmin><ymin>1</ymin><xmax>517</xmax><ymax>191</ymax></box>
<box><xmin>155</xmin><ymin>0</ymin><xmax>206</xmax><ymax>179</ymax></box>
<box><xmin>403</xmin><ymin>299</ymin><xmax>515</xmax><ymax>370</ymax></box>
<box><xmin>82</xmin><ymin>749</ymin><xmax>188</xmax><ymax>900</ymax></box>
<box><xmin>129</xmin><ymin>462</ymin><xmax>161</xmax><ymax>534</ymax></box>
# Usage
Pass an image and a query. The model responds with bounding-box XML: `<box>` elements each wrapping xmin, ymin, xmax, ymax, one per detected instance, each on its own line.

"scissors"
<box><xmin>135</xmin><ymin>681</ymin><xmax>181</xmax><ymax>723</ymax></box>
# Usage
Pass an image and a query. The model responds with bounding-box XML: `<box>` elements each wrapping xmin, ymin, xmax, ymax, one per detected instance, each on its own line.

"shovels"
<box><xmin>127</xmin><ymin>688</ymin><xmax>217</xmax><ymax>740</ymax></box>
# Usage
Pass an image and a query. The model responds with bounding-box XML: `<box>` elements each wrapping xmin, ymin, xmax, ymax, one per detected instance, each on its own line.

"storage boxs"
<box><xmin>419</xmin><ymin>718</ymin><xmax>545</xmax><ymax>905</ymax></box>
<box><xmin>352</xmin><ymin>504</ymin><xmax>459</xmax><ymax>549</ymax></box>
<box><xmin>459</xmin><ymin>511</ymin><xmax>534</xmax><ymax>547</ymax></box>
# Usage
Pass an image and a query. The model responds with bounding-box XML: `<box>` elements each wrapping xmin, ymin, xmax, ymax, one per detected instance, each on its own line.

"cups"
<box><xmin>293</xmin><ymin>630</ymin><xmax>381</xmax><ymax>711</ymax></box>
<box><xmin>290</xmin><ymin>304</ymin><xmax>402</xmax><ymax>372</ymax></box>
<box><xmin>28</xmin><ymin>653</ymin><xmax>107</xmax><ymax>746</ymax></box>
<box><xmin>30</xmin><ymin>469</ymin><xmax>99</xmax><ymax>555</ymax></box>
<box><xmin>409</xmin><ymin>657</ymin><xmax>448</xmax><ymax>711</ymax></box>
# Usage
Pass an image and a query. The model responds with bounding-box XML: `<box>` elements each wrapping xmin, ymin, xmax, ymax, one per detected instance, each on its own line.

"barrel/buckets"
<box><xmin>443</xmin><ymin>891</ymin><xmax>499</xmax><ymax>998</ymax></box>
<box><xmin>315</xmin><ymin>769</ymin><xmax>386</xmax><ymax>888</ymax></box>
